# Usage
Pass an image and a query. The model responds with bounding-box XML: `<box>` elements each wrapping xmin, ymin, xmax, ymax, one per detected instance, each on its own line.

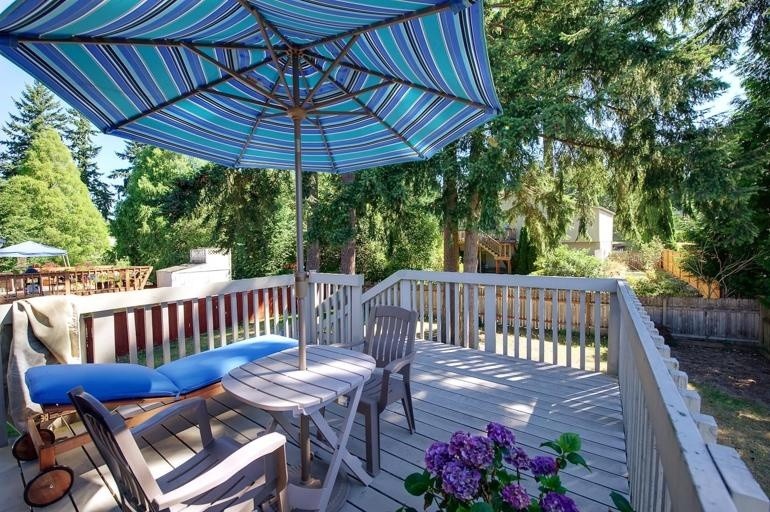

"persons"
<box><xmin>24</xmin><ymin>266</ymin><xmax>40</xmax><ymax>284</ymax></box>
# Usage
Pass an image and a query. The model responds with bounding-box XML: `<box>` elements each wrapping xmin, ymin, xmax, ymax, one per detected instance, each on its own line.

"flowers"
<box><xmin>395</xmin><ymin>420</ymin><xmax>592</xmax><ymax>512</ymax></box>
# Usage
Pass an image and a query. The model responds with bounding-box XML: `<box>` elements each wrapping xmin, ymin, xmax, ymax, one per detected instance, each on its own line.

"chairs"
<box><xmin>12</xmin><ymin>334</ymin><xmax>299</xmax><ymax>508</ymax></box>
<box><xmin>67</xmin><ymin>385</ymin><xmax>289</xmax><ymax>512</ymax></box>
<box><xmin>317</xmin><ymin>304</ymin><xmax>419</xmax><ymax>477</ymax></box>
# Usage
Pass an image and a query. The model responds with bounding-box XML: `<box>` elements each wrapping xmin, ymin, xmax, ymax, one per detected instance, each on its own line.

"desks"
<box><xmin>221</xmin><ymin>344</ymin><xmax>376</xmax><ymax>512</ymax></box>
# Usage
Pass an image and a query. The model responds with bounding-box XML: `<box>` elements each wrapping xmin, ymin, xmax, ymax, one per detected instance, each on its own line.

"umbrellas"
<box><xmin>0</xmin><ymin>0</ymin><xmax>503</xmax><ymax>480</ymax></box>
